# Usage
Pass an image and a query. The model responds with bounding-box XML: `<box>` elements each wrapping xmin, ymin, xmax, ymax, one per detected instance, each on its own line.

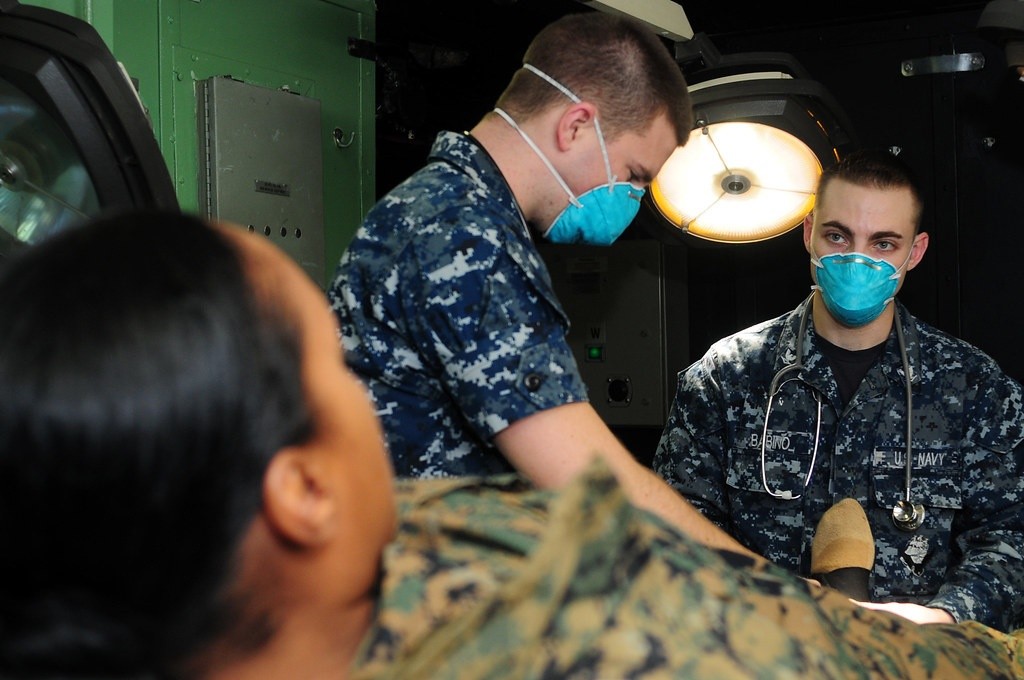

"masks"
<box><xmin>809</xmin><ymin>235</ymin><xmax>915</xmax><ymax>329</ymax></box>
<box><xmin>492</xmin><ymin>64</ymin><xmax>647</xmax><ymax>249</ymax></box>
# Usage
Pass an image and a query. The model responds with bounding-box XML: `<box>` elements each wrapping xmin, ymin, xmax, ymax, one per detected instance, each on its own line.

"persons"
<box><xmin>324</xmin><ymin>0</ymin><xmax>821</xmax><ymax>588</ymax></box>
<box><xmin>0</xmin><ymin>210</ymin><xmax>1024</xmax><ymax>680</ymax></box>
<box><xmin>653</xmin><ymin>149</ymin><xmax>1024</xmax><ymax>634</ymax></box>
